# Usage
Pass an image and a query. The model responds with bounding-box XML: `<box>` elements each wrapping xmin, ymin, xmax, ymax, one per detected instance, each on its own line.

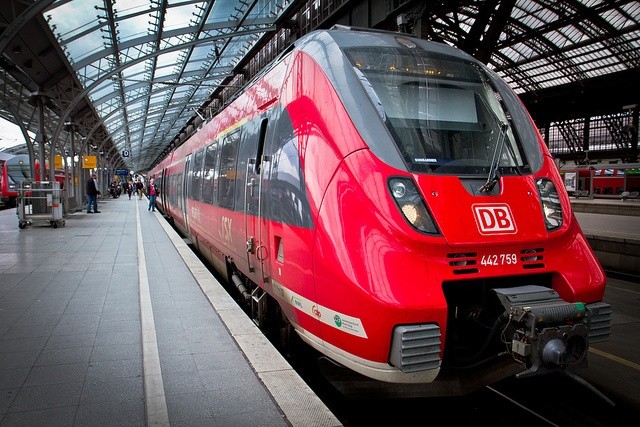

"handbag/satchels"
<box><xmin>155</xmin><ymin>188</ymin><xmax>160</xmax><ymax>197</ymax></box>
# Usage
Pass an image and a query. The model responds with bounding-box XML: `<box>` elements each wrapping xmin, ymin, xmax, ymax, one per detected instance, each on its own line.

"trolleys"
<box><xmin>14</xmin><ymin>179</ymin><xmax>66</xmax><ymax>231</ymax></box>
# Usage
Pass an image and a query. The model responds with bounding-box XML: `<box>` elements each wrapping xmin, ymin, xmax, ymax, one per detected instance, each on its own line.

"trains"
<box><xmin>145</xmin><ymin>23</ymin><xmax>612</xmax><ymax>405</ymax></box>
<box><xmin>1</xmin><ymin>153</ymin><xmax>70</xmax><ymax>206</ymax></box>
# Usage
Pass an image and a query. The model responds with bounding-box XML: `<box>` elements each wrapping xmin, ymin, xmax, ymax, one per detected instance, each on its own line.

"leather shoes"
<box><xmin>87</xmin><ymin>210</ymin><xmax>93</xmax><ymax>213</ymax></box>
<box><xmin>94</xmin><ymin>210</ymin><xmax>101</xmax><ymax>213</ymax></box>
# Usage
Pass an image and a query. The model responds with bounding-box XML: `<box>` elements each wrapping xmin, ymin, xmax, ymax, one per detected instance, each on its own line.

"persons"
<box><xmin>109</xmin><ymin>174</ymin><xmax>144</xmax><ymax>201</ymax></box>
<box><xmin>86</xmin><ymin>173</ymin><xmax>102</xmax><ymax>214</ymax></box>
<box><xmin>147</xmin><ymin>178</ymin><xmax>158</xmax><ymax>212</ymax></box>
